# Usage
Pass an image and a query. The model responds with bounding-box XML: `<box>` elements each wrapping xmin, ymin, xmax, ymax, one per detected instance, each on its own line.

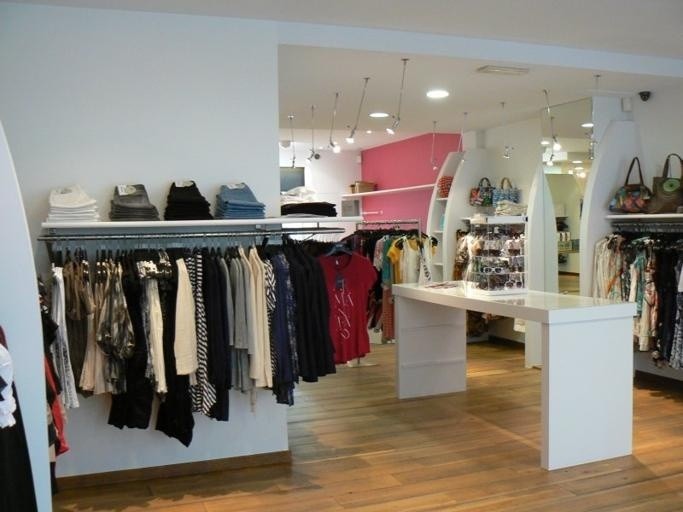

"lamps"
<box><xmin>329</xmin><ymin>93</ymin><xmax>342</xmax><ymax>154</ymax></box>
<box><xmin>567</xmin><ymin>130</ymin><xmax>592</xmax><ymax>179</ymax></box>
<box><xmin>306</xmin><ymin>106</ymin><xmax>316</xmax><ymax>163</ymax></box>
<box><xmin>386</xmin><ymin>58</ymin><xmax>408</xmax><ymax>136</ymax></box>
<box><xmin>542</xmin><ymin>88</ymin><xmax>561</xmax><ymax>166</ymax></box>
<box><xmin>503</xmin><ymin>100</ymin><xmax>511</xmax><ymax>158</ymax></box>
<box><xmin>346</xmin><ymin>78</ymin><xmax>369</xmax><ymax>143</ymax></box>
<box><xmin>288</xmin><ymin>115</ymin><xmax>296</xmax><ymax>170</ymax></box>
<box><xmin>430</xmin><ymin>119</ymin><xmax>439</xmax><ymax>172</ymax></box>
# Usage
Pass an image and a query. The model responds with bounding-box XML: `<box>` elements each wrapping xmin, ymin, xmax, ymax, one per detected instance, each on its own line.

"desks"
<box><xmin>392</xmin><ymin>280</ymin><xmax>637</xmax><ymax>470</ymax></box>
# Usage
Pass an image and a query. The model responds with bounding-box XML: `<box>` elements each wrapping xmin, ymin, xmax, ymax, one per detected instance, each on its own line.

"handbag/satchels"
<box><xmin>651</xmin><ymin>153</ymin><xmax>683</xmax><ymax>215</ymax></box>
<box><xmin>608</xmin><ymin>158</ymin><xmax>653</xmax><ymax>214</ymax></box>
<box><xmin>491</xmin><ymin>177</ymin><xmax>520</xmax><ymax>207</ymax></box>
<box><xmin>470</xmin><ymin>177</ymin><xmax>496</xmax><ymax>208</ymax></box>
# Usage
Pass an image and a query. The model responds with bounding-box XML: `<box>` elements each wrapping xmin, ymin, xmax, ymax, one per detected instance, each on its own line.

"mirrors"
<box><xmin>541</xmin><ymin>98</ymin><xmax>592</xmax><ymax>297</ymax></box>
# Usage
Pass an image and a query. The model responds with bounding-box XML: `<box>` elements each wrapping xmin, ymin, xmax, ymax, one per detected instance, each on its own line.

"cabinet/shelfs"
<box><xmin>426</xmin><ymin>149</ymin><xmax>482</xmax><ymax>283</ymax></box>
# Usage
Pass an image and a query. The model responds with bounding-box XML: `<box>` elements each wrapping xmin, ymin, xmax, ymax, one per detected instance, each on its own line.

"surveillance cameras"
<box><xmin>638</xmin><ymin>91</ymin><xmax>652</xmax><ymax>102</ymax></box>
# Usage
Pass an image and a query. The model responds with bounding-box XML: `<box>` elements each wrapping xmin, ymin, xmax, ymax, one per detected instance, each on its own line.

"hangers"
<box><xmin>607</xmin><ymin>223</ymin><xmax>683</xmax><ymax>251</ymax></box>
<box><xmin>358</xmin><ymin>220</ymin><xmax>438</xmax><ymax>248</ymax></box>
<box><xmin>53</xmin><ymin>230</ymin><xmax>354</xmax><ymax>268</ymax></box>
